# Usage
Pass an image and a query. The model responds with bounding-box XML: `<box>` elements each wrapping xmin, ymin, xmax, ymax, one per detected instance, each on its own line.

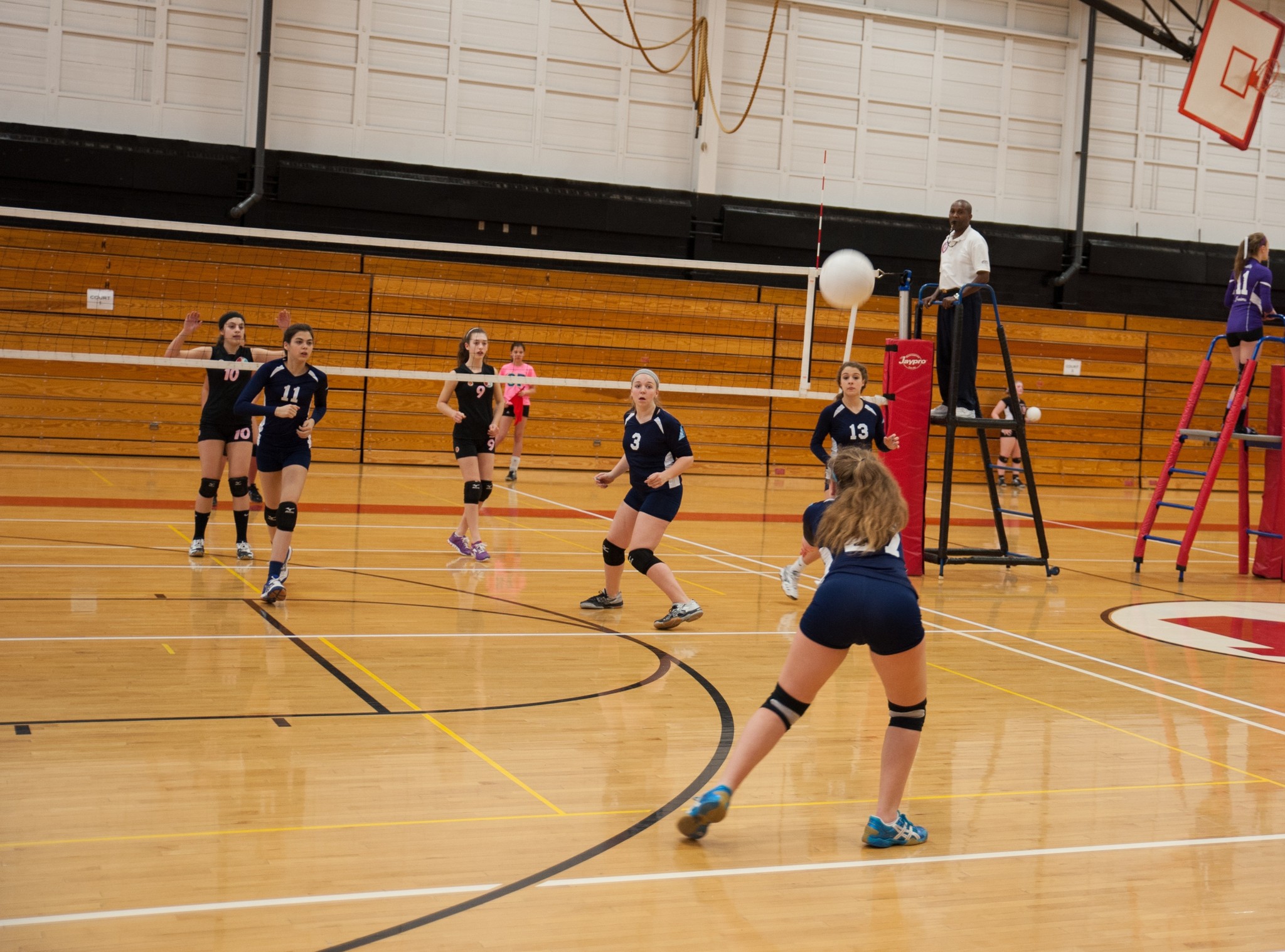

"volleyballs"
<box><xmin>1027</xmin><ymin>406</ymin><xmax>1041</xmax><ymax>421</ymax></box>
<box><xmin>819</xmin><ymin>250</ymin><xmax>875</xmax><ymax>308</ymax></box>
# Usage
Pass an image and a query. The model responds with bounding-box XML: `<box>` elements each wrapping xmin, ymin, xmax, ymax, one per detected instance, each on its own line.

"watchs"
<box><xmin>953</xmin><ymin>293</ymin><xmax>961</xmax><ymax>301</ymax></box>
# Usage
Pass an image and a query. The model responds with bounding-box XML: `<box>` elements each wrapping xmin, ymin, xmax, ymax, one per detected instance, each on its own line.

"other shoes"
<box><xmin>955</xmin><ymin>407</ymin><xmax>976</xmax><ymax>418</ymax></box>
<box><xmin>999</xmin><ymin>477</ymin><xmax>1007</xmax><ymax>486</ymax></box>
<box><xmin>1012</xmin><ymin>477</ymin><xmax>1025</xmax><ymax>487</ymax></box>
<box><xmin>779</xmin><ymin>564</ymin><xmax>800</xmax><ymax>600</ymax></box>
<box><xmin>505</xmin><ymin>469</ymin><xmax>517</xmax><ymax>481</ymax></box>
<box><xmin>1233</xmin><ymin>426</ymin><xmax>1258</xmax><ymax>435</ymax></box>
<box><xmin>930</xmin><ymin>404</ymin><xmax>947</xmax><ymax>416</ymax></box>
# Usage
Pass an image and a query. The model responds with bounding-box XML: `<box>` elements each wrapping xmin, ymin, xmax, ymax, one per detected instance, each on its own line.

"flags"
<box><xmin>510</xmin><ymin>386</ymin><xmax>523</xmax><ymax>426</ymax></box>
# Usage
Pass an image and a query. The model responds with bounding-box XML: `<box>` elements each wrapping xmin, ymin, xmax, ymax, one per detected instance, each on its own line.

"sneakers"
<box><xmin>235</xmin><ymin>560</ymin><xmax>254</xmax><ymax>573</ymax></box>
<box><xmin>446</xmin><ymin>556</ymin><xmax>492</xmax><ymax>580</ymax></box>
<box><xmin>261</xmin><ymin>575</ymin><xmax>287</xmax><ymax>603</ymax></box>
<box><xmin>677</xmin><ymin>784</ymin><xmax>732</xmax><ymax>839</ymax></box>
<box><xmin>579</xmin><ymin>587</ymin><xmax>623</xmax><ymax>609</ymax></box>
<box><xmin>447</xmin><ymin>531</ymin><xmax>471</xmax><ymax>556</ymax></box>
<box><xmin>471</xmin><ymin>541</ymin><xmax>490</xmax><ymax>562</ymax></box>
<box><xmin>861</xmin><ymin>809</ymin><xmax>927</xmax><ymax>848</ymax></box>
<box><xmin>267</xmin><ymin>545</ymin><xmax>292</xmax><ymax>583</ymax></box>
<box><xmin>654</xmin><ymin>598</ymin><xmax>703</xmax><ymax>629</ymax></box>
<box><xmin>189</xmin><ymin>539</ymin><xmax>205</xmax><ymax>557</ymax></box>
<box><xmin>236</xmin><ymin>541</ymin><xmax>253</xmax><ymax>559</ymax></box>
<box><xmin>188</xmin><ymin>557</ymin><xmax>205</xmax><ymax>572</ymax></box>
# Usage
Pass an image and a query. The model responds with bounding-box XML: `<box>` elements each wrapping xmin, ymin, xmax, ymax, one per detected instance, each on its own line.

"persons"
<box><xmin>677</xmin><ymin>447</ymin><xmax>928</xmax><ymax>848</ymax></box>
<box><xmin>779</xmin><ymin>362</ymin><xmax>899</xmax><ymax>601</ymax></box>
<box><xmin>165</xmin><ymin>309</ymin><xmax>328</xmax><ymax>606</ymax></box>
<box><xmin>1222</xmin><ymin>231</ymin><xmax>1278</xmax><ymax>434</ymax></box>
<box><xmin>493</xmin><ymin>340</ymin><xmax>537</xmax><ymax>483</ymax></box>
<box><xmin>923</xmin><ymin>200</ymin><xmax>991</xmax><ymax>417</ymax></box>
<box><xmin>580</xmin><ymin>368</ymin><xmax>703</xmax><ymax>630</ymax></box>
<box><xmin>435</xmin><ymin>326</ymin><xmax>503</xmax><ymax>562</ymax></box>
<box><xmin>990</xmin><ymin>380</ymin><xmax>1034</xmax><ymax>488</ymax></box>
